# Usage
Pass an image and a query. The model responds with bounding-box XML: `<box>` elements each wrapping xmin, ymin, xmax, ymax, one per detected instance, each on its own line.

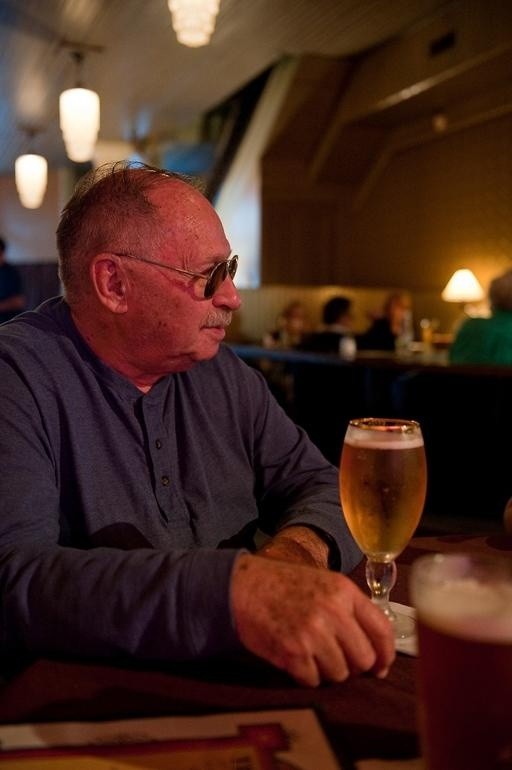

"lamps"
<box><xmin>13</xmin><ymin>39</ymin><xmax>103</xmax><ymax>211</ymax></box>
<box><xmin>441</xmin><ymin>269</ymin><xmax>485</xmax><ymax>319</ymax></box>
<box><xmin>168</xmin><ymin>1</ymin><xmax>221</xmax><ymax>48</ymax></box>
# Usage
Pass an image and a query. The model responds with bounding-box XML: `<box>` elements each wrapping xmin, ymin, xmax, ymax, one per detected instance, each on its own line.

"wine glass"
<box><xmin>337</xmin><ymin>415</ymin><xmax>428</xmax><ymax>642</ymax></box>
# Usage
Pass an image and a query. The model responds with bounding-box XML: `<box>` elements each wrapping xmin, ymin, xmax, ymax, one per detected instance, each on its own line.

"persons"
<box><xmin>0</xmin><ymin>158</ymin><xmax>397</xmax><ymax>688</ymax></box>
<box><xmin>369</xmin><ymin>293</ymin><xmax>410</xmax><ymax>354</ymax></box>
<box><xmin>264</xmin><ymin>301</ymin><xmax>311</xmax><ymax>352</ymax></box>
<box><xmin>448</xmin><ymin>267</ymin><xmax>512</xmax><ymax>366</ymax></box>
<box><xmin>306</xmin><ymin>295</ymin><xmax>359</xmax><ymax>357</ymax></box>
<box><xmin>0</xmin><ymin>237</ymin><xmax>28</xmax><ymax>326</ymax></box>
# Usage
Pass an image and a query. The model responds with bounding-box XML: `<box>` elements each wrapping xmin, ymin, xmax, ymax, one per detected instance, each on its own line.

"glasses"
<box><xmin>105</xmin><ymin>254</ymin><xmax>238</xmax><ymax>298</ymax></box>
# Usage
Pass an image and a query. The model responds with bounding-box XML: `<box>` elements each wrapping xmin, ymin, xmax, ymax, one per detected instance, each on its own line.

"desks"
<box><xmin>1</xmin><ymin>532</ymin><xmax>512</xmax><ymax>769</ymax></box>
